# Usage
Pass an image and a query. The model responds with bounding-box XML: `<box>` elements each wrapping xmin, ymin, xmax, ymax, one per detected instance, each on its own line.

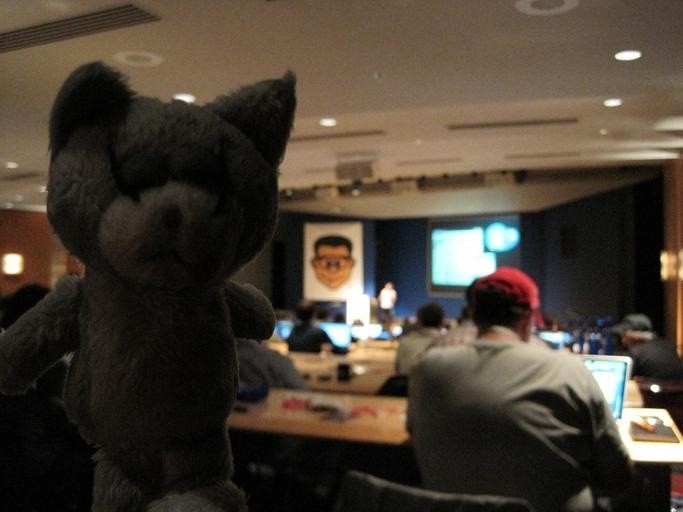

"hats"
<box><xmin>607</xmin><ymin>313</ymin><xmax>652</xmax><ymax>336</ymax></box>
<box><xmin>474</xmin><ymin>267</ymin><xmax>545</xmax><ymax>328</ymax></box>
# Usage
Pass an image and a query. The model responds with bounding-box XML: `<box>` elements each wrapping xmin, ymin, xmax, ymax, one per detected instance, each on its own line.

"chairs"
<box><xmin>343</xmin><ymin>468</ymin><xmax>531</xmax><ymax>511</ymax></box>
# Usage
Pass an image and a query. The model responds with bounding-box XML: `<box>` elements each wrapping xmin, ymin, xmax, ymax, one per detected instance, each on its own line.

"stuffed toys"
<box><xmin>0</xmin><ymin>60</ymin><xmax>296</xmax><ymax>512</ymax></box>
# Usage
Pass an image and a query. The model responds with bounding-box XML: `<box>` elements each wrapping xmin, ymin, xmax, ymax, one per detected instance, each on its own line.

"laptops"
<box><xmin>537</xmin><ymin>326</ymin><xmax>565</xmax><ymax>354</ymax></box>
<box><xmin>274</xmin><ymin>319</ymin><xmax>385</xmax><ymax>351</ymax></box>
<box><xmin>570</xmin><ymin>354</ymin><xmax>634</xmax><ymax>426</ymax></box>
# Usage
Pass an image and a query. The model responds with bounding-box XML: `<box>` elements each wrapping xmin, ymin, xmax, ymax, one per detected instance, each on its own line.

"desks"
<box><xmin>225</xmin><ymin>341</ymin><xmax>412</xmax><ymax>474</ymax></box>
<box><xmin>616</xmin><ymin>379</ymin><xmax>683</xmax><ymax>509</ymax></box>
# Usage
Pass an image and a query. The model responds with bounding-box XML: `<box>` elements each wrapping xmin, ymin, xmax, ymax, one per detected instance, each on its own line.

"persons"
<box><xmin>311</xmin><ymin>236</ymin><xmax>354</xmax><ymax>287</ymax></box>
